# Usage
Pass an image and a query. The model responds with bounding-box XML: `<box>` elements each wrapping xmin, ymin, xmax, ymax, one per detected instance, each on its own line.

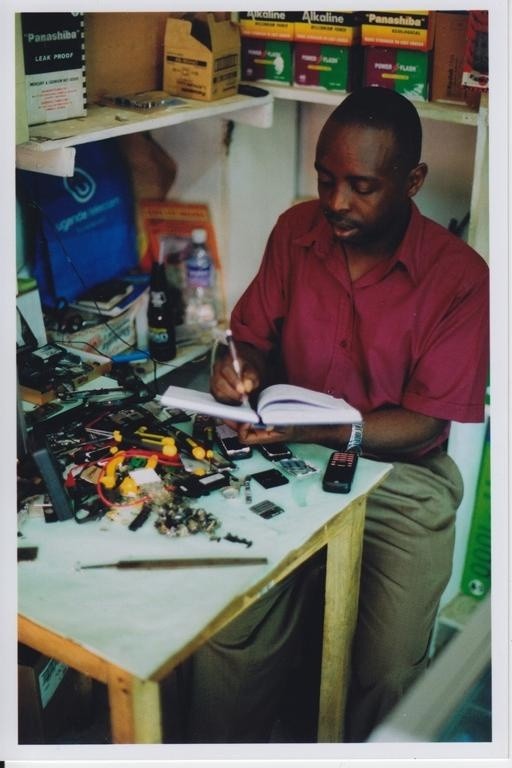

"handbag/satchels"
<box><xmin>15</xmin><ymin>140</ymin><xmax>142</xmax><ymax>312</ymax></box>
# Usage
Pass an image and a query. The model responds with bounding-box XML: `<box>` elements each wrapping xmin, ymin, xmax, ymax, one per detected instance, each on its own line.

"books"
<box><xmin>135</xmin><ymin>198</ymin><xmax>227</xmax><ymax>325</ymax></box>
<box><xmin>70</xmin><ymin>279</ymin><xmax>134</xmax><ymax>311</ymax></box>
<box><xmin>19</xmin><ymin>12</ymin><xmax>88</xmax><ymax>127</ymax></box>
<box><xmin>161</xmin><ymin>380</ymin><xmax>365</xmax><ymax>429</ymax></box>
<box><xmin>20</xmin><ymin>343</ymin><xmax>113</xmax><ymax>406</ymax></box>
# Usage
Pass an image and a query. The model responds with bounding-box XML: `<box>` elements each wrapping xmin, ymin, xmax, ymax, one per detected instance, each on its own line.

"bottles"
<box><xmin>147</xmin><ymin>229</ymin><xmax>218</xmax><ymax>361</ymax></box>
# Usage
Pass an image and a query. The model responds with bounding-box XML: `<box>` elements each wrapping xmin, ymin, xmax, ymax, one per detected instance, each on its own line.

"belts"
<box><xmin>411</xmin><ymin>438</ymin><xmax>449</xmax><ymax>469</ymax></box>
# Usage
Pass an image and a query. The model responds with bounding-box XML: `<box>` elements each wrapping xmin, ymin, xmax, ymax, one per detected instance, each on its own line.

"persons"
<box><xmin>186</xmin><ymin>88</ymin><xmax>490</xmax><ymax>744</ymax></box>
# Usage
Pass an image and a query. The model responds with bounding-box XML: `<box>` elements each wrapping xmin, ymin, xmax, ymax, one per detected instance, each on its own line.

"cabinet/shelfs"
<box><xmin>18</xmin><ymin>85</ymin><xmax>275</xmax><ymax>386</ymax></box>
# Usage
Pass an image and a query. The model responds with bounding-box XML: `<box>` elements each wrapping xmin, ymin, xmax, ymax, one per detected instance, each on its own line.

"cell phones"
<box><xmin>322</xmin><ymin>451</ymin><xmax>358</xmax><ymax>493</ymax></box>
<box><xmin>215</xmin><ymin>424</ymin><xmax>252</xmax><ymax>459</ymax></box>
<box><xmin>260</xmin><ymin>443</ymin><xmax>292</xmax><ymax>462</ymax></box>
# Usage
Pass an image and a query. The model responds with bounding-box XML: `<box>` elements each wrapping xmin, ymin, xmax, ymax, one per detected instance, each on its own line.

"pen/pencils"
<box><xmin>225</xmin><ymin>329</ymin><xmax>249</xmax><ymax>409</ymax></box>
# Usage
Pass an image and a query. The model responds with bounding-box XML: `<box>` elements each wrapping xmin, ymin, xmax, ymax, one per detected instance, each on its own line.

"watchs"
<box><xmin>344</xmin><ymin>421</ymin><xmax>364</xmax><ymax>457</ymax></box>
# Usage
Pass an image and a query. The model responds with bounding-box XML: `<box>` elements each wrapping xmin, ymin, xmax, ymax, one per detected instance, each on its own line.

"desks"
<box><xmin>19</xmin><ymin>422</ymin><xmax>390</xmax><ymax>744</ymax></box>
